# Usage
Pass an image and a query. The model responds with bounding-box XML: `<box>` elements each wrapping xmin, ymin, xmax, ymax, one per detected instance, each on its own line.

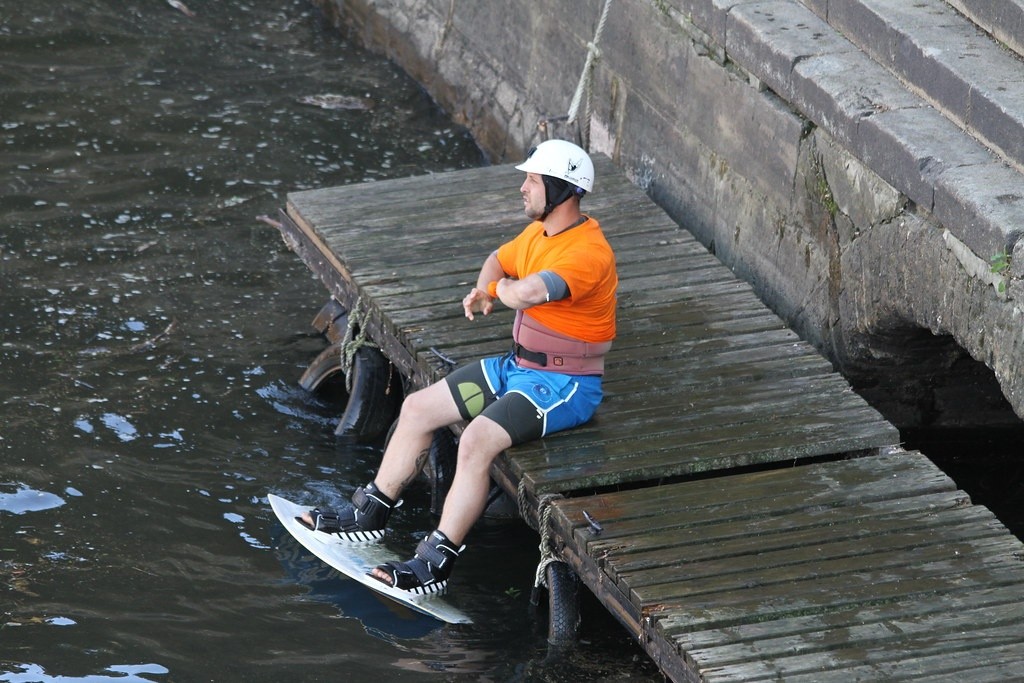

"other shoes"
<box><xmin>305</xmin><ymin>483</ymin><xmax>396</xmax><ymax>536</ymax></box>
<box><xmin>368</xmin><ymin>530</ymin><xmax>466</xmax><ymax>596</ymax></box>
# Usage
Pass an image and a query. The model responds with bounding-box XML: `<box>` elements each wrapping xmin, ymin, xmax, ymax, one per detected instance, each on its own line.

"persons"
<box><xmin>300</xmin><ymin>139</ymin><xmax>618</xmax><ymax>596</ymax></box>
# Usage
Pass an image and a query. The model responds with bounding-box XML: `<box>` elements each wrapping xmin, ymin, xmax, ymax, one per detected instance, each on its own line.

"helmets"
<box><xmin>515</xmin><ymin>138</ymin><xmax>594</xmax><ymax>192</ymax></box>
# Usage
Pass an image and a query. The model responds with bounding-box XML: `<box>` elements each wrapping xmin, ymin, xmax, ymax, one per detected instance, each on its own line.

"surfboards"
<box><xmin>265</xmin><ymin>490</ymin><xmax>475</xmax><ymax>624</ymax></box>
